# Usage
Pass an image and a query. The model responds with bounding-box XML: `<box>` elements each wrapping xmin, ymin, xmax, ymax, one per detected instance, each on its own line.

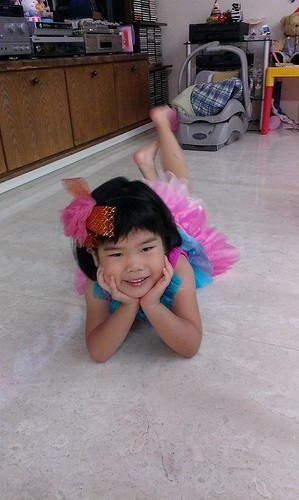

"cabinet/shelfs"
<box><xmin>0</xmin><ymin>59</ymin><xmax>72</xmax><ymax>181</ymax></box>
<box><xmin>113</xmin><ymin>54</ymin><xmax>152</xmax><ymax>134</ymax></box>
<box><xmin>183</xmin><ymin>38</ymin><xmax>272</xmax><ymax>132</ymax></box>
<box><xmin>65</xmin><ymin>57</ymin><xmax>120</xmax><ymax>153</ymax></box>
<box><xmin>117</xmin><ymin>0</ymin><xmax>170</xmax><ymax>114</ymax></box>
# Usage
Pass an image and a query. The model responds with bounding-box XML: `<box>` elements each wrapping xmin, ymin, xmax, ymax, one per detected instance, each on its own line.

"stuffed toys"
<box><xmin>270</xmin><ymin>12</ymin><xmax>299</xmax><ymax>65</ymax></box>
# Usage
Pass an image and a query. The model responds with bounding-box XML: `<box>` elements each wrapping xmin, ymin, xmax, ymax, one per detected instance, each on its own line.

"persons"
<box><xmin>85</xmin><ymin>106</ymin><xmax>204</xmax><ymax>363</ymax></box>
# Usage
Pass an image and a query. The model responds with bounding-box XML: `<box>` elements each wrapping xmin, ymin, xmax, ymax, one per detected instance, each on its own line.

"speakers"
<box><xmin>31</xmin><ymin>37</ymin><xmax>86</xmax><ymax>57</ymax></box>
<box><xmin>188</xmin><ymin>22</ymin><xmax>250</xmax><ymax>43</ymax></box>
<box><xmin>196</xmin><ymin>53</ymin><xmax>255</xmax><ymax>67</ymax></box>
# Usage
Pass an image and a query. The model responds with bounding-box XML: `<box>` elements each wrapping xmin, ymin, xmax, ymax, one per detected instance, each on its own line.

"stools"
<box><xmin>261</xmin><ymin>65</ymin><xmax>299</xmax><ymax>134</ymax></box>
